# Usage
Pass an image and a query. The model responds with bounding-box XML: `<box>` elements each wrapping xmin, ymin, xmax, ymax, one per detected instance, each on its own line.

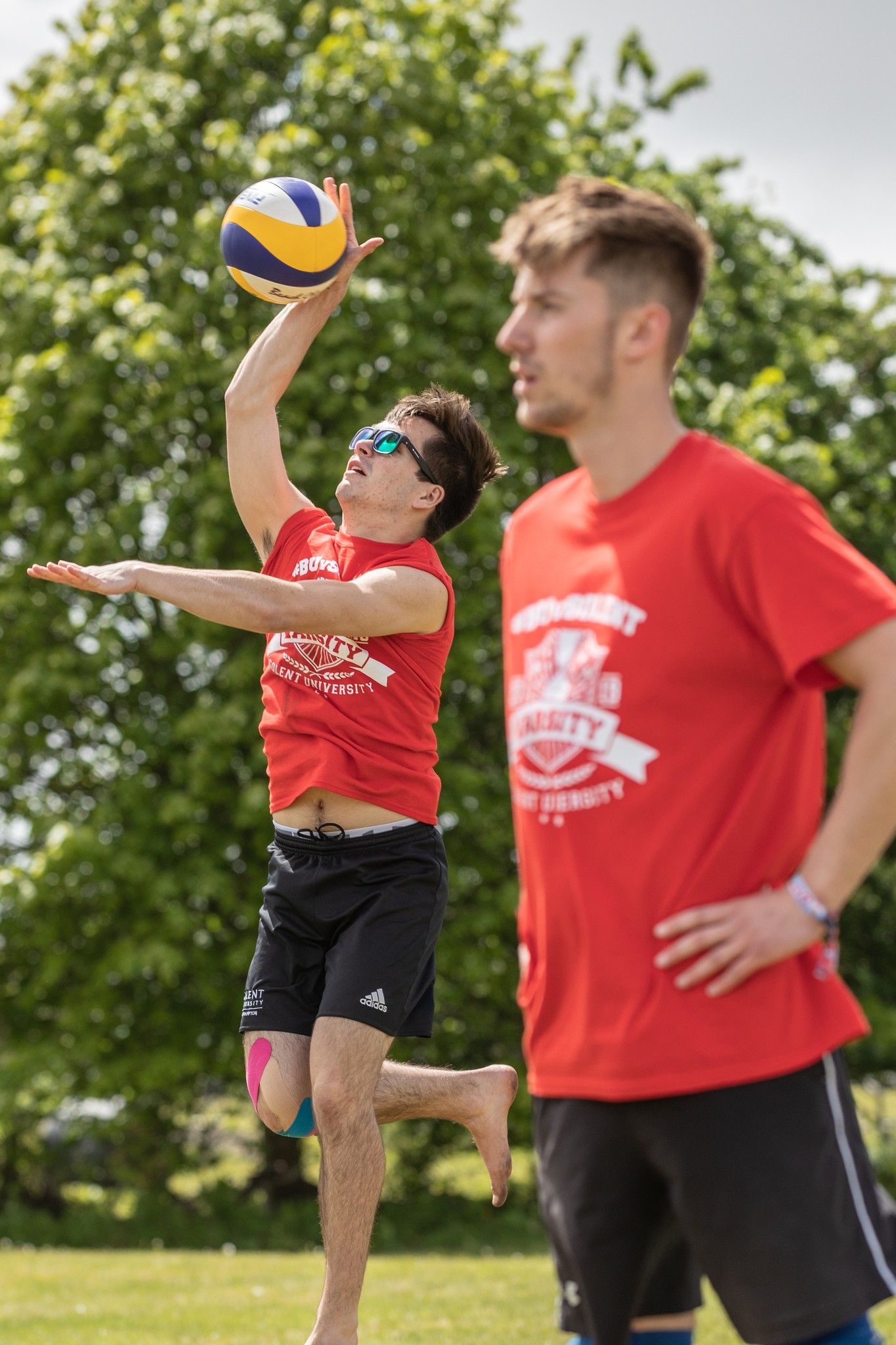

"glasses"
<box><xmin>348</xmin><ymin>426</ymin><xmax>441</xmax><ymax>512</ymax></box>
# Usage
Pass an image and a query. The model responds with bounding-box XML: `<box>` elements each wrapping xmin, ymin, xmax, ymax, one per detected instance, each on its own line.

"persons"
<box><xmin>28</xmin><ymin>178</ymin><xmax>521</xmax><ymax>1345</ymax></box>
<box><xmin>484</xmin><ymin>175</ymin><xmax>896</xmax><ymax>1345</ymax></box>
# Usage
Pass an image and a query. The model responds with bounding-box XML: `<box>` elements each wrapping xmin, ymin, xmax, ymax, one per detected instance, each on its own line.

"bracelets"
<box><xmin>787</xmin><ymin>874</ymin><xmax>840</xmax><ymax>944</ymax></box>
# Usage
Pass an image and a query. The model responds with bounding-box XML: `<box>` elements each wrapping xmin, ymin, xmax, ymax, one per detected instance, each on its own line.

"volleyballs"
<box><xmin>220</xmin><ymin>177</ymin><xmax>348</xmax><ymax>305</ymax></box>
